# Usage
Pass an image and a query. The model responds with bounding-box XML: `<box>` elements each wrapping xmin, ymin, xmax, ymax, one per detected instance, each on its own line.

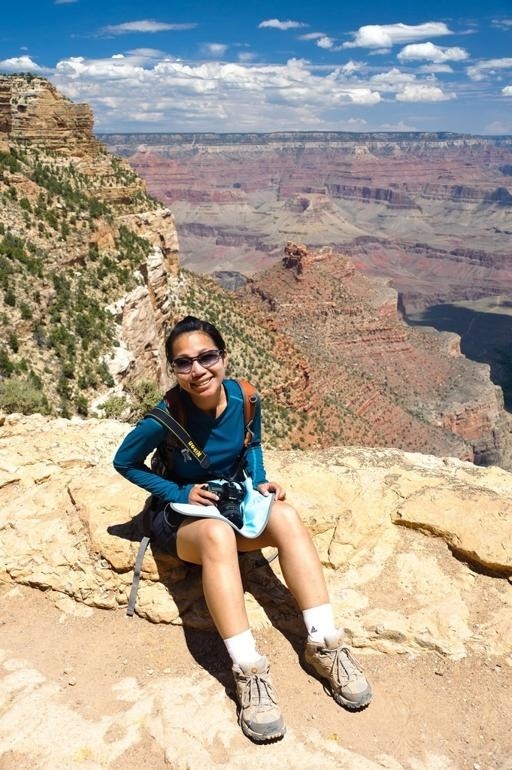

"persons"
<box><xmin>113</xmin><ymin>316</ymin><xmax>372</xmax><ymax>741</ymax></box>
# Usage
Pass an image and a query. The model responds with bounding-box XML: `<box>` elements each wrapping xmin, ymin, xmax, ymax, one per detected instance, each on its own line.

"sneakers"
<box><xmin>231</xmin><ymin>654</ymin><xmax>286</xmax><ymax>741</ymax></box>
<box><xmin>304</xmin><ymin>628</ymin><xmax>373</xmax><ymax>709</ymax></box>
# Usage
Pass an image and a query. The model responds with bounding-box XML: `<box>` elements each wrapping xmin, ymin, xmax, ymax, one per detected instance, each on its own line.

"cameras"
<box><xmin>201</xmin><ymin>479</ymin><xmax>247</xmax><ymax>530</ymax></box>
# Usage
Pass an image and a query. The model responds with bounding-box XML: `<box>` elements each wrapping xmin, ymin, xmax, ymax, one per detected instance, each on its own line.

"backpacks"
<box><xmin>151</xmin><ymin>379</ymin><xmax>258</xmax><ymax>480</ymax></box>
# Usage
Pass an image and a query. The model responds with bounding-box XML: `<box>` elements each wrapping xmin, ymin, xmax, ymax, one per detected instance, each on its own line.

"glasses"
<box><xmin>173</xmin><ymin>350</ymin><xmax>223</xmax><ymax>374</ymax></box>
